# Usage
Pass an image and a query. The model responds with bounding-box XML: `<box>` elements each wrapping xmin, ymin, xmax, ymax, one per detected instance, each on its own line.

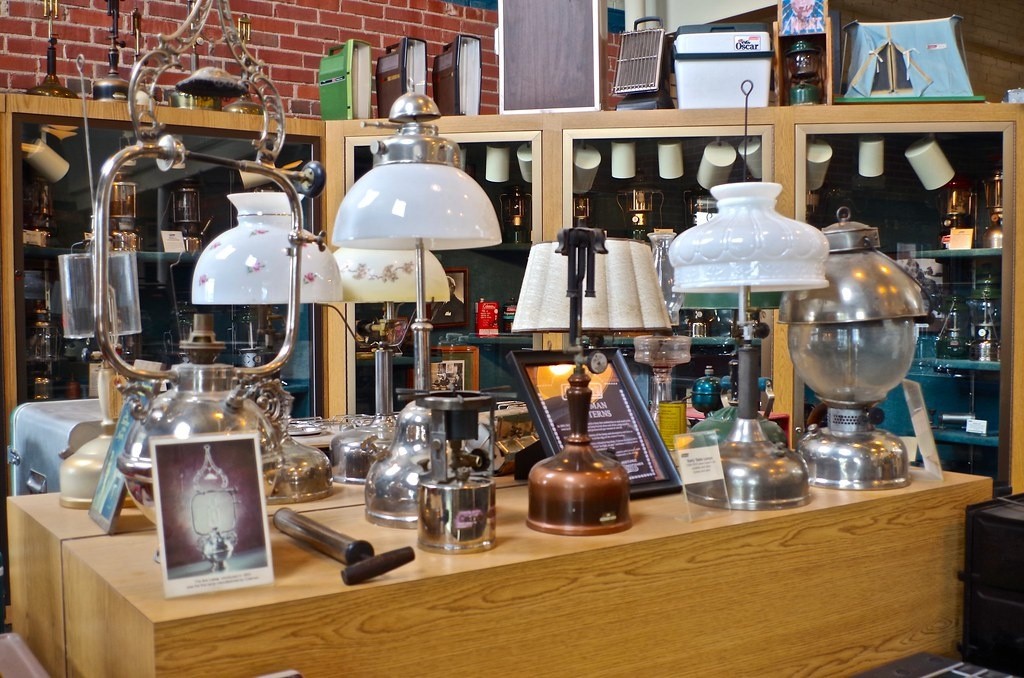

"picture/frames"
<box><xmin>406</xmin><ymin>344</ymin><xmax>480</xmax><ymax>400</ymax></box>
<box><xmin>505</xmin><ymin>346</ymin><xmax>686</xmax><ymax>499</ymax></box>
<box><xmin>426</xmin><ymin>266</ymin><xmax>469</xmax><ymax>328</ymax></box>
<box><xmin>148</xmin><ymin>430</ymin><xmax>275</xmax><ymax>599</ymax></box>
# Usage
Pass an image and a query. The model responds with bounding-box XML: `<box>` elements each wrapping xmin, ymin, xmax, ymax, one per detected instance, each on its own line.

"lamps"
<box><xmin>806</xmin><ymin>134</ymin><xmax>833</xmax><ymax>191</ymax></box>
<box><xmin>773</xmin><ymin>206</ymin><xmax>927</xmax><ymax>493</ymax></box>
<box><xmin>903</xmin><ymin>132</ymin><xmax>955</xmax><ymax>191</ymax></box>
<box><xmin>330</xmin><ymin>245</ymin><xmax>451</xmax><ymax>483</ymax></box>
<box><xmin>485</xmin><ymin>142</ymin><xmax>509</xmax><ymax>182</ymax></box>
<box><xmin>517</xmin><ymin>142</ymin><xmax>532</xmax><ymax>183</ymax></box>
<box><xmin>858</xmin><ymin>134</ymin><xmax>884</xmax><ymax>178</ymax></box>
<box><xmin>696</xmin><ymin>136</ymin><xmax>738</xmax><ymax>190</ymax></box>
<box><xmin>737</xmin><ymin>136</ymin><xmax>763</xmax><ymax>180</ymax></box>
<box><xmin>666</xmin><ymin>181</ymin><xmax>830</xmax><ymax>512</ymax></box>
<box><xmin>785</xmin><ymin>40</ymin><xmax>824</xmax><ymax>108</ymax></box>
<box><xmin>189</xmin><ymin>188</ymin><xmax>345</xmax><ymax>507</ymax></box>
<box><xmin>510</xmin><ymin>237</ymin><xmax>671</xmax><ymax>537</ymax></box>
<box><xmin>332</xmin><ymin>76</ymin><xmax>504</xmax><ymax>531</ymax></box>
<box><xmin>572</xmin><ymin>139</ymin><xmax>602</xmax><ymax>194</ymax></box>
<box><xmin>610</xmin><ymin>138</ymin><xmax>636</xmax><ymax>179</ymax></box>
<box><xmin>657</xmin><ymin>138</ymin><xmax>684</xmax><ymax>179</ymax></box>
<box><xmin>632</xmin><ymin>229</ymin><xmax>693</xmax><ymax>421</ymax></box>
<box><xmin>24</xmin><ymin>138</ymin><xmax>70</xmax><ymax>184</ymax></box>
<box><xmin>56</xmin><ymin>234</ymin><xmax>146</xmax><ymax>511</ymax></box>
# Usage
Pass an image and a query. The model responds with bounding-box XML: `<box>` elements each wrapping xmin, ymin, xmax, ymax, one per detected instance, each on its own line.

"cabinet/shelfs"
<box><xmin>0</xmin><ymin>92</ymin><xmax>329</xmax><ymax>500</ymax></box>
<box><xmin>324</xmin><ymin>99</ymin><xmax>1024</xmax><ymax>502</ymax></box>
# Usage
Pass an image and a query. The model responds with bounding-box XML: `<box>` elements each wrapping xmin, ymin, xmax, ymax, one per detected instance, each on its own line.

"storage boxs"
<box><xmin>671</xmin><ymin>22</ymin><xmax>776</xmax><ymax>109</ymax></box>
<box><xmin>474</xmin><ymin>302</ymin><xmax>499</xmax><ymax>338</ymax></box>
